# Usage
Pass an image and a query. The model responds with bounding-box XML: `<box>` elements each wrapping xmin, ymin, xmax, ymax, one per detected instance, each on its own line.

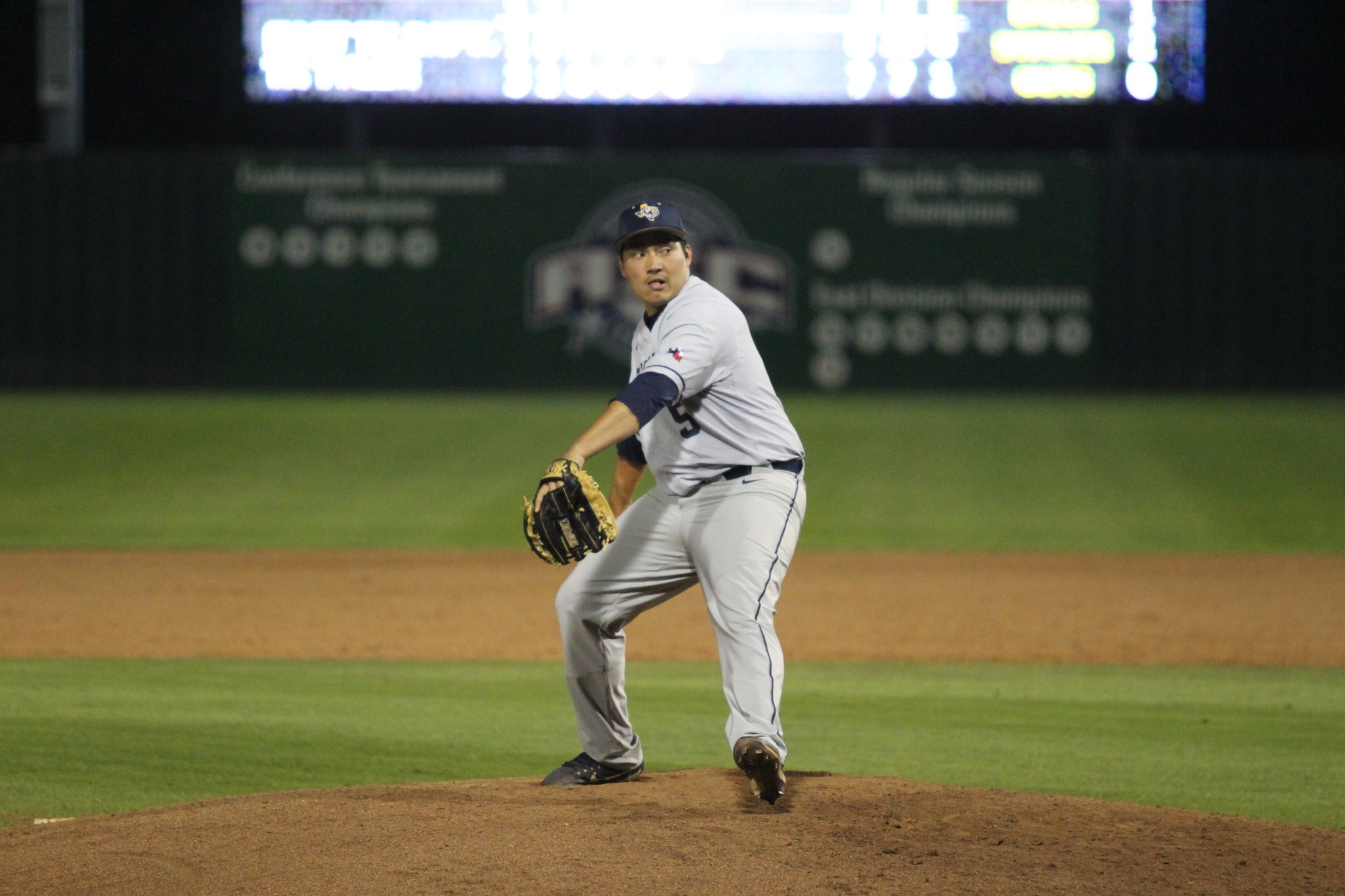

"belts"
<box><xmin>722</xmin><ymin>459</ymin><xmax>804</xmax><ymax>480</ymax></box>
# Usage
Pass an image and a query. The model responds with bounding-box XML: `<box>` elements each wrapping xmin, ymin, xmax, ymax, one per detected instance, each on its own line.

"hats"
<box><xmin>614</xmin><ymin>201</ymin><xmax>687</xmax><ymax>256</ymax></box>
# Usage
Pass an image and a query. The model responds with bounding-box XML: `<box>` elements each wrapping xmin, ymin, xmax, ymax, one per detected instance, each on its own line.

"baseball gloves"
<box><xmin>520</xmin><ymin>459</ymin><xmax>618</xmax><ymax>566</ymax></box>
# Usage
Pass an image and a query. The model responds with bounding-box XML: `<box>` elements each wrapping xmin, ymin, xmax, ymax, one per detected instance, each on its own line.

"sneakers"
<box><xmin>540</xmin><ymin>752</ymin><xmax>644</xmax><ymax>786</ymax></box>
<box><xmin>733</xmin><ymin>738</ymin><xmax>785</xmax><ymax>805</ymax></box>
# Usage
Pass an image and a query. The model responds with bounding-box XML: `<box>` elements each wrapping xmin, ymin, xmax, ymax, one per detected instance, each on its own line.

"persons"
<box><xmin>536</xmin><ymin>201</ymin><xmax>808</xmax><ymax>806</ymax></box>
